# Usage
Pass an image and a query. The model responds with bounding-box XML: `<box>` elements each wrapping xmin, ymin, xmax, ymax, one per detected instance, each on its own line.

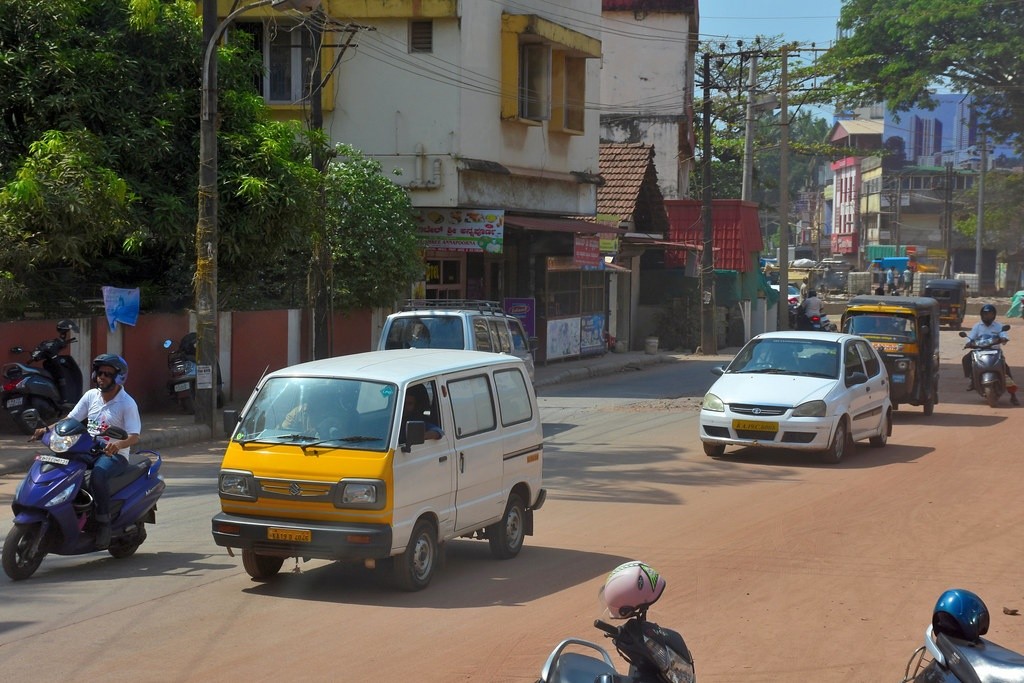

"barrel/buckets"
<box><xmin>644</xmin><ymin>336</ymin><xmax>658</xmax><ymax>354</ymax></box>
<box><xmin>611</xmin><ymin>337</ymin><xmax>629</xmax><ymax>353</ymax></box>
<box><xmin>72</xmin><ymin>488</ymin><xmax>93</xmax><ymax>535</ymax></box>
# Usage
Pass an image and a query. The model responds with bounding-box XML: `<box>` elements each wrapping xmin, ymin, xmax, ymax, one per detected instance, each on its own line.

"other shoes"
<box><xmin>95</xmin><ymin>525</ymin><xmax>112</xmax><ymax>549</ymax></box>
<box><xmin>1011</xmin><ymin>399</ymin><xmax>1021</xmax><ymax>406</ymax></box>
<box><xmin>967</xmin><ymin>384</ymin><xmax>974</xmax><ymax>391</ymax></box>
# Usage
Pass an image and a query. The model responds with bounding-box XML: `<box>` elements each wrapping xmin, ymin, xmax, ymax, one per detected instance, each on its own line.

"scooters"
<box><xmin>0</xmin><ymin>322</ymin><xmax>84</xmax><ymax>435</ymax></box>
<box><xmin>163</xmin><ymin>332</ymin><xmax>225</xmax><ymax>415</ymax></box>
<box><xmin>1</xmin><ymin>408</ymin><xmax>166</xmax><ymax>583</ymax></box>
<box><xmin>958</xmin><ymin>324</ymin><xmax>1011</xmax><ymax>408</ymax></box>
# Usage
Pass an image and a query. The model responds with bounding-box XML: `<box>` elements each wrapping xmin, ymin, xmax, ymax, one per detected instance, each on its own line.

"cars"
<box><xmin>699</xmin><ymin>330</ymin><xmax>893</xmax><ymax>464</ymax></box>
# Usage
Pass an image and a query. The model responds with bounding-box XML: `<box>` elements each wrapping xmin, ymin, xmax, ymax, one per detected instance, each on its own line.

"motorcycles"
<box><xmin>796</xmin><ymin>307</ymin><xmax>837</xmax><ymax>332</ymax></box>
<box><xmin>534</xmin><ymin>604</ymin><xmax>697</xmax><ymax>683</ymax></box>
<box><xmin>924</xmin><ymin>279</ymin><xmax>969</xmax><ymax>330</ymax></box>
<box><xmin>840</xmin><ymin>295</ymin><xmax>941</xmax><ymax>416</ymax></box>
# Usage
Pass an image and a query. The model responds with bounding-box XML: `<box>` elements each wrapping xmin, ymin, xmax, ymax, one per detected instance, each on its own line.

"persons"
<box><xmin>801</xmin><ymin>277</ymin><xmax>809</xmax><ymax>301</ymax></box>
<box><xmin>389</xmin><ymin>382</ymin><xmax>445</xmax><ymax>440</ymax></box>
<box><xmin>798</xmin><ymin>290</ymin><xmax>824</xmax><ymax>330</ymax></box>
<box><xmin>962</xmin><ymin>305</ymin><xmax>1020</xmax><ymax>405</ymax></box>
<box><xmin>30</xmin><ymin>352</ymin><xmax>141</xmax><ymax>549</ymax></box>
<box><xmin>281</xmin><ymin>392</ymin><xmax>332</xmax><ymax>436</ymax></box>
<box><xmin>879</xmin><ymin>266</ymin><xmax>913</xmax><ymax>293</ymax></box>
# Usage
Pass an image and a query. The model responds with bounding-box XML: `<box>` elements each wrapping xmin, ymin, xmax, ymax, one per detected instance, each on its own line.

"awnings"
<box><xmin>504</xmin><ymin>214</ymin><xmax>629</xmax><ymax>234</ymax></box>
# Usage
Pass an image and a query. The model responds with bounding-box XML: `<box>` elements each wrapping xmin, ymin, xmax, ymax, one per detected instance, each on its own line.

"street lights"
<box><xmin>701</xmin><ymin>94</ymin><xmax>779</xmax><ymax>357</ymax></box>
<box><xmin>195</xmin><ymin>0</ymin><xmax>294</xmax><ymax>424</ymax></box>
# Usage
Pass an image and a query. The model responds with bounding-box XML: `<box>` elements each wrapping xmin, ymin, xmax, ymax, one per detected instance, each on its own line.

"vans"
<box><xmin>211</xmin><ymin>347</ymin><xmax>546</xmax><ymax>592</ymax></box>
<box><xmin>376</xmin><ymin>299</ymin><xmax>539</xmax><ymax>399</ymax></box>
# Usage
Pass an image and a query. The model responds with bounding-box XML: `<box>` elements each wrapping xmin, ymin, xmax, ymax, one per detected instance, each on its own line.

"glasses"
<box><xmin>97</xmin><ymin>371</ymin><xmax>115</xmax><ymax>377</ymax></box>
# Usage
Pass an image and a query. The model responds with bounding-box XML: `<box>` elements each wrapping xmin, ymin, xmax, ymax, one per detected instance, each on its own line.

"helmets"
<box><xmin>980</xmin><ymin>305</ymin><xmax>997</xmax><ymax>325</ymax></box>
<box><xmin>931</xmin><ymin>589</ymin><xmax>990</xmax><ymax>641</ymax></box>
<box><xmin>598</xmin><ymin>561</ymin><xmax>666</xmax><ymax>619</ymax></box>
<box><xmin>92</xmin><ymin>354</ymin><xmax>128</xmax><ymax>387</ymax></box>
